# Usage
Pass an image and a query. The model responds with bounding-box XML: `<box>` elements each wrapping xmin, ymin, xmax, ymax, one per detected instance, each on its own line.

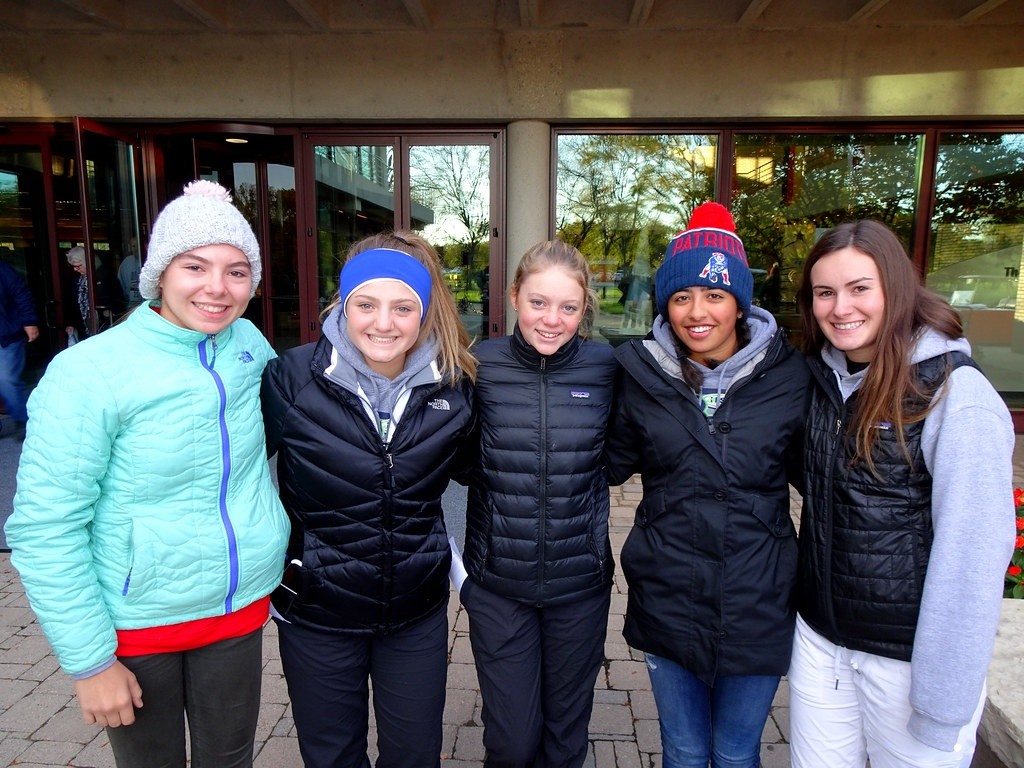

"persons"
<box><xmin>787</xmin><ymin>221</ymin><xmax>1016</xmax><ymax>768</ymax></box>
<box><xmin>65</xmin><ymin>246</ymin><xmax>127</xmax><ymax>337</ymax></box>
<box><xmin>459</xmin><ymin>240</ymin><xmax>617</xmax><ymax>768</ymax></box>
<box><xmin>117</xmin><ymin>236</ymin><xmax>145</xmax><ymax>308</ymax></box>
<box><xmin>3</xmin><ymin>179</ymin><xmax>293</xmax><ymax>768</ymax></box>
<box><xmin>606</xmin><ymin>201</ymin><xmax>812</xmax><ymax>768</ymax></box>
<box><xmin>259</xmin><ymin>230</ymin><xmax>476</xmax><ymax>768</ymax></box>
<box><xmin>0</xmin><ymin>262</ymin><xmax>37</xmax><ymax>440</ymax></box>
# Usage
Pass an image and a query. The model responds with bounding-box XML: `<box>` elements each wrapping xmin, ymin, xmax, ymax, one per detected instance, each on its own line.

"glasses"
<box><xmin>74</xmin><ymin>265</ymin><xmax>82</xmax><ymax>269</ymax></box>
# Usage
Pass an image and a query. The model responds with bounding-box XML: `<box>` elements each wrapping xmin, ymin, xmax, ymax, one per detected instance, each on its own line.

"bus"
<box><xmin>950</xmin><ymin>276</ymin><xmax>1017</xmax><ymax>307</ymax></box>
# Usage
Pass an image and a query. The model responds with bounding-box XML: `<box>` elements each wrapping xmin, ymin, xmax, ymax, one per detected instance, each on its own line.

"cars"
<box><xmin>953</xmin><ymin>298</ymin><xmax>1016</xmax><ymax>310</ymax></box>
<box><xmin>594</xmin><ymin>271</ymin><xmax>625</xmax><ymax>281</ymax></box>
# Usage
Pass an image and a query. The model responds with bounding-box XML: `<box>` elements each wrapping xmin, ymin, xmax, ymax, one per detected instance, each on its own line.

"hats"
<box><xmin>138</xmin><ymin>180</ymin><xmax>262</xmax><ymax>300</ymax></box>
<box><xmin>655</xmin><ymin>202</ymin><xmax>753</xmax><ymax>322</ymax></box>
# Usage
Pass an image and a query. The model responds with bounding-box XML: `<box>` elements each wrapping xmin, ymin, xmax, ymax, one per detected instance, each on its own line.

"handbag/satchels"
<box><xmin>68</xmin><ymin>329</ymin><xmax>79</xmax><ymax>348</ymax></box>
